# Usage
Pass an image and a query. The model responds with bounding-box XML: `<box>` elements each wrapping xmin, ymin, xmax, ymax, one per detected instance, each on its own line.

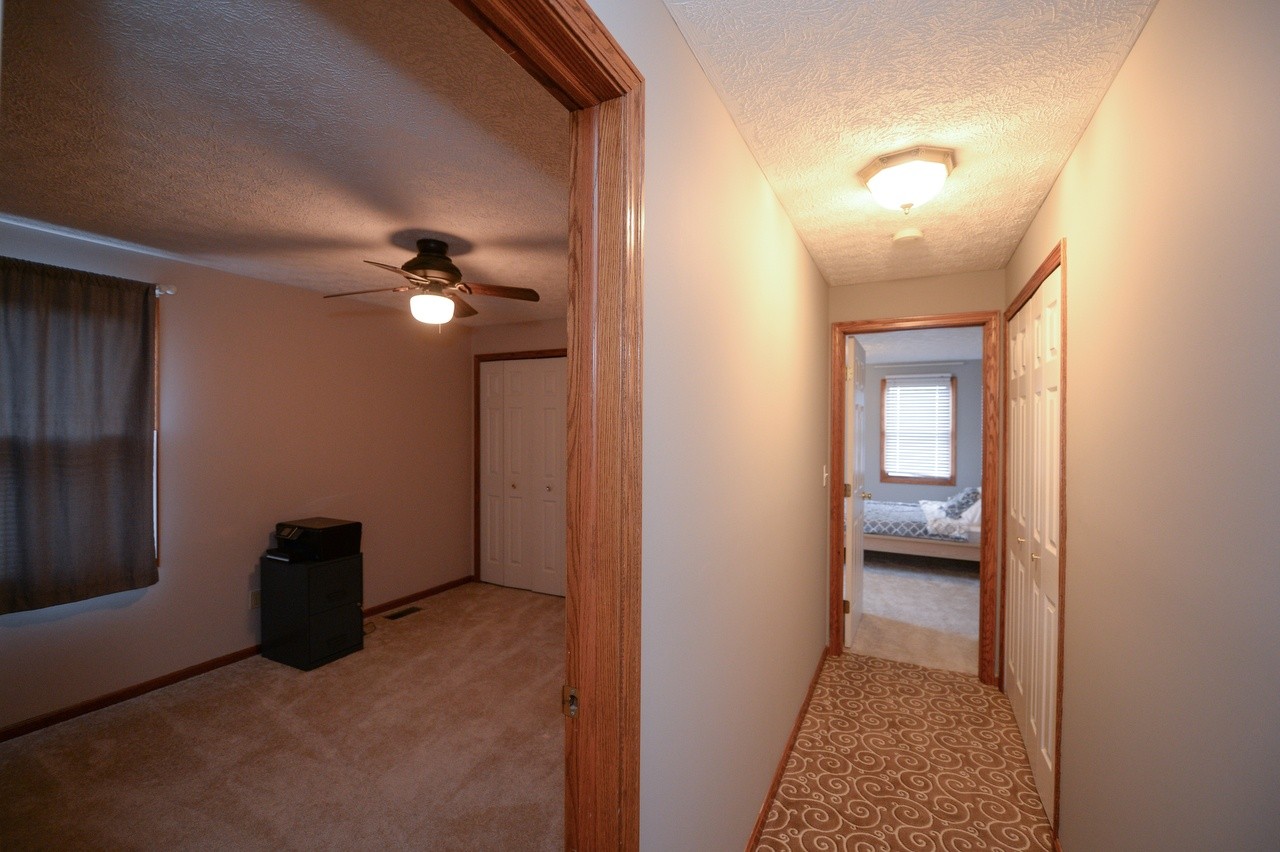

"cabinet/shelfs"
<box><xmin>261</xmin><ymin>550</ymin><xmax>364</xmax><ymax>671</ymax></box>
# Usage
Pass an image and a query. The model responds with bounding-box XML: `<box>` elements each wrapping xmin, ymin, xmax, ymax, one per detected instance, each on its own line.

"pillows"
<box><xmin>941</xmin><ymin>485</ymin><xmax>979</xmax><ymax>517</ymax></box>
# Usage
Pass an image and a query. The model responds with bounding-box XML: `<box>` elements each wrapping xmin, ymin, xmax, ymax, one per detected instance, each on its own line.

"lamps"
<box><xmin>857</xmin><ymin>145</ymin><xmax>960</xmax><ymax>248</ymax></box>
<box><xmin>408</xmin><ymin>283</ymin><xmax>456</xmax><ymax>326</ymax></box>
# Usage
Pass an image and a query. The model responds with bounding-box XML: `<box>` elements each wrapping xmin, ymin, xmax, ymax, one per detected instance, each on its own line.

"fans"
<box><xmin>322</xmin><ymin>235</ymin><xmax>542</xmax><ymax>326</ymax></box>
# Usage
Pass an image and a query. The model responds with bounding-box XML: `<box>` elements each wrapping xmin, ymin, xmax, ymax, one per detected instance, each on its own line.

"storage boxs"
<box><xmin>274</xmin><ymin>516</ymin><xmax>362</xmax><ymax>559</ymax></box>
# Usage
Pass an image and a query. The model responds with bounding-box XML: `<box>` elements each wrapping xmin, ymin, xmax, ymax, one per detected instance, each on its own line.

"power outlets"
<box><xmin>248</xmin><ymin>588</ymin><xmax>261</xmax><ymax>611</ymax></box>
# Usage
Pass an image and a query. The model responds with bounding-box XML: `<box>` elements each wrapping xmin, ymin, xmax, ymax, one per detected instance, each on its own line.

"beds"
<box><xmin>863</xmin><ymin>487</ymin><xmax>981</xmax><ymax>563</ymax></box>
